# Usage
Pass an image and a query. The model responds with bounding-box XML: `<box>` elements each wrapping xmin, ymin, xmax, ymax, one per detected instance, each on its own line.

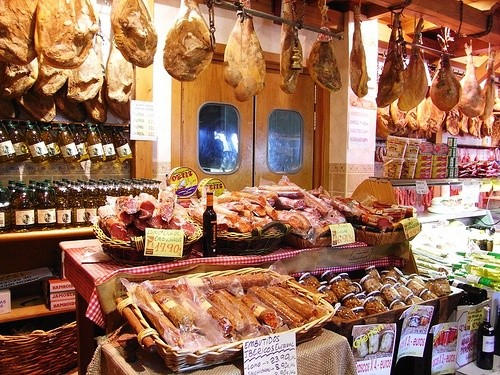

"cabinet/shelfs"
<box><xmin>0</xmin><ymin>118</ymin><xmax>163</xmax><ymax>327</ymax></box>
<box><xmin>409</xmin><ymin>207</ymin><xmax>493</xmax><ymax>230</ymax></box>
<box><xmin>374</xmin><ymin>131</ymin><xmax>438</xmax><ymax>176</ymax></box>
<box><xmin>439</xmin><ymin>112</ymin><xmax>500</xmax><ymax>180</ymax></box>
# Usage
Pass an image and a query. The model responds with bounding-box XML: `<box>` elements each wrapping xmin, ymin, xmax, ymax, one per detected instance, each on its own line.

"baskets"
<box><xmin>287</xmin><ymin>234</ymin><xmax>331</xmax><ymax>248</ymax></box>
<box><xmin>354</xmin><ymin>229</ymin><xmax>406</xmax><ymax>246</ymax></box>
<box><xmin>0</xmin><ymin>320</ymin><xmax>78</xmax><ymax>375</ymax></box>
<box><xmin>113</xmin><ymin>268</ymin><xmax>335</xmax><ymax>373</ymax></box>
<box><xmin>216</xmin><ymin>221</ymin><xmax>291</xmax><ymax>255</ymax></box>
<box><xmin>93</xmin><ymin>213</ymin><xmax>203</xmax><ymax>265</ymax></box>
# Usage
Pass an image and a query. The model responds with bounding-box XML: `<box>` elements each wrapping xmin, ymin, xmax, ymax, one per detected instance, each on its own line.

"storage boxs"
<box><xmin>327</xmin><ymin>275</ymin><xmax>465</xmax><ymax>348</ymax></box>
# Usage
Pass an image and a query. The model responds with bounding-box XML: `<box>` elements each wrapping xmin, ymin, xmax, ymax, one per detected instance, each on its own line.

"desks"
<box><xmin>59</xmin><ymin>230</ymin><xmax>406</xmax><ymax>375</ymax></box>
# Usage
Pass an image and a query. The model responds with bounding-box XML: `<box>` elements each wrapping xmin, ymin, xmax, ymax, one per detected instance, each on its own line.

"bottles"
<box><xmin>0</xmin><ymin>178</ymin><xmax>162</xmax><ymax>234</ymax></box>
<box><xmin>494</xmin><ymin>304</ymin><xmax>500</xmax><ymax>356</ymax></box>
<box><xmin>203</xmin><ymin>192</ymin><xmax>217</xmax><ymax>258</ymax></box>
<box><xmin>447</xmin><ymin>279</ymin><xmax>487</xmax><ymax>304</ymax></box>
<box><xmin>477</xmin><ymin>307</ymin><xmax>495</xmax><ymax>370</ymax></box>
<box><xmin>0</xmin><ymin>119</ymin><xmax>133</xmax><ymax>165</ymax></box>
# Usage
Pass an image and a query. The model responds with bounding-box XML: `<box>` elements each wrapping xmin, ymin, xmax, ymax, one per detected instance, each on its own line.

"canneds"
<box><xmin>298</xmin><ymin>265</ymin><xmax>452</xmax><ymax>320</ymax></box>
